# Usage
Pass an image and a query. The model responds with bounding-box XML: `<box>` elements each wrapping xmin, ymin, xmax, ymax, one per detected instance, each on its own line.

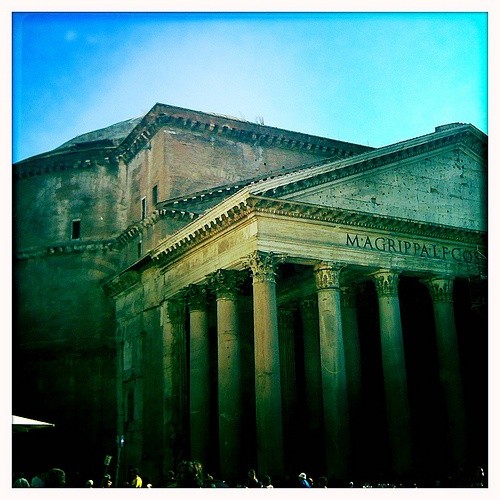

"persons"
<box><xmin>13</xmin><ymin>459</ymin><xmax>421</xmax><ymax>487</ymax></box>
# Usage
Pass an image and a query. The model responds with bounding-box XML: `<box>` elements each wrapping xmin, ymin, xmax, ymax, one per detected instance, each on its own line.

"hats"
<box><xmin>299</xmin><ymin>473</ymin><xmax>306</xmax><ymax>480</ymax></box>
